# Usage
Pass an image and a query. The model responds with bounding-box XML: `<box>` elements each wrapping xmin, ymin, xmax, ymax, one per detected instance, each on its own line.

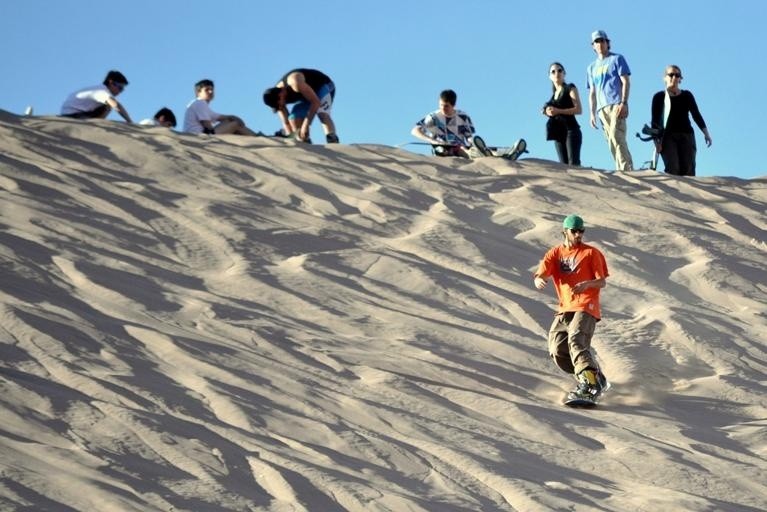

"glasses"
<box><xmin>567</xmin><ymin>228</ymin><xmax>585</xmax><ymax>234</ymax></box>
<box><xmin>665</xmin><ymin>73</ymin><xmax>681</xmax><ymax>77</ymax></box>
<box><xmin>549</xmin><ymin>69</ymin><xmax>564</xmax><ymax>74</ymax></box>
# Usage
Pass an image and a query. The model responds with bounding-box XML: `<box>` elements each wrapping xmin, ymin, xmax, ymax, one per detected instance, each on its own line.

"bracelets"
<box><xmin>620</xmin><ymin>101</ymin><xmax>627</xmax><ymax>105</ymax></box>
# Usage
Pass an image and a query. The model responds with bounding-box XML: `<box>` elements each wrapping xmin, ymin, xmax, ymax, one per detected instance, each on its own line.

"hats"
<box><xmin>562</xmin><ymin>214</ymin><xmax>585</xmax><ymax>230</ymax></box>
<box><xmin>590</xmin><ymin>30</ymin><xmax>611</xmax><ymax>44</ymax></box>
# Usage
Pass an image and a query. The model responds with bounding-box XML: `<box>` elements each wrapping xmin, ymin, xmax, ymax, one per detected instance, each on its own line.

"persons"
<box><xmin>649</xmin><ymin>63</ymin><xmax>711</xmax><ymax>177</ymax></box>
<box><xmin>137</xmin><ymin>106</ymin><xmax>176</xmax><ymax>127</ymax></box>
<box><xmin>411</xmin><ymin>88</ymin><xmax>475</xmax><ymax>160</ymax></box>
<box><xmin>533</xmin><ymin>215</ymin><xmax>609</xmax><ymax>410</ymax></box>
<box><xmin>181</xmin><ymin>80</ymin><xmax>258</xmax><ymax>137</ymax></box>
<box><xmin>56</xmin><ymin>72</ymin><xmax>135</xmax><ymax>122</ymax></box>
<box><xmin>584</xmin><ymin>29</ymin><xmax>635</xmax><ymax>173</ymax></box>
<box><xmin>542</xmin><ymin>63</ymin><xmax>581</xmax><ymax>167</ymax></box>
<box><xmin>261</xmin><ymin>67</ymin><xmax>339</xmax><ymax>143</ymax></box>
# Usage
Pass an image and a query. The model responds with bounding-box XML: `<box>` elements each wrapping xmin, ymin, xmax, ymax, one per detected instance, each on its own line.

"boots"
<box><xmin>564</xmin><ymin>366</ymin><xmax>611</xmax><ymax>406</ymax></box>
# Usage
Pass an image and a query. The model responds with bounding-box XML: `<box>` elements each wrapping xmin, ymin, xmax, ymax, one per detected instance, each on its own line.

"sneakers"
<box><xmin>508</xmin><ymin>138</ymin><xmax>526</xmax><ymax>160</ymax></box>
<box><xmin>304</xmin><ymin>138</ymin><xmax>311</xmax><ymax>144</ymax></box>
<box><xmin>325</xmin><ymin>133</ymin><xmax>338</xmax><ymax>143</ymax></box>
<box><xmin>473</xmin><ymin>135</ymin><xmax>494</xmax><ymax>156</ymax></box>
